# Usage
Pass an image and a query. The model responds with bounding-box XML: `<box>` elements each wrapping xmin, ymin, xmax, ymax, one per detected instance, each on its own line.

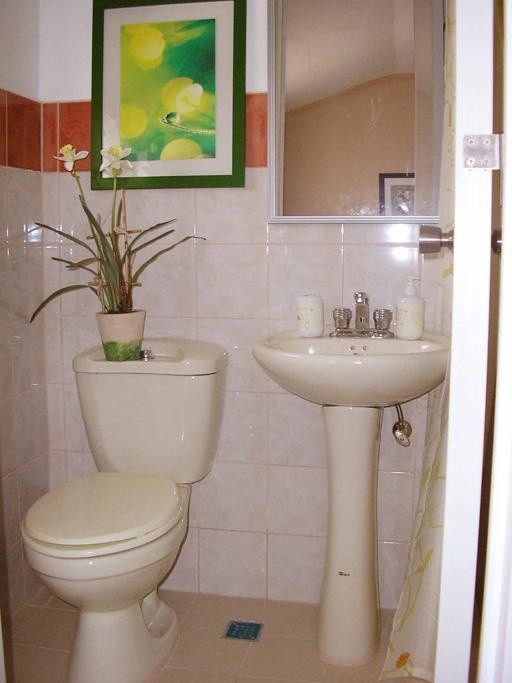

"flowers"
<box><xmin>26</xmin><ymin>137</ymin><xmax>206</xmax><ymax>325</ymax></box>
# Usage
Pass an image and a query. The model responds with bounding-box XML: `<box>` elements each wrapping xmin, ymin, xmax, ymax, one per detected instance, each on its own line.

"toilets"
<box><xmin>20</xmin><ymin>337</ymin><xmax>227</xmax><ymax>683</ymax></box>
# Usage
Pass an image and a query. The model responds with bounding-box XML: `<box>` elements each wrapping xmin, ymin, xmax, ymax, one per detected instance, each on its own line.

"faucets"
<box><xmin>353</xmin><ymin>291</ymin><xmax>369</xmax><ymax>337</ymax></box>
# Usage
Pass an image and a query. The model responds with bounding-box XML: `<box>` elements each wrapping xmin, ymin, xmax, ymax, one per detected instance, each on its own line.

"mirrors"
<box><xmin>262</xmin><ymin>0</ymin><xmax>447</xmax><ymax>225</ymax></box>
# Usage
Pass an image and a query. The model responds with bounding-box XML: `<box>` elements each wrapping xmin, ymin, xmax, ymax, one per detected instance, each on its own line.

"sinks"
<box><xmin>252</xmin><ymin>327</ymin><xmax>448</xmax><ymax>406</ymax></box>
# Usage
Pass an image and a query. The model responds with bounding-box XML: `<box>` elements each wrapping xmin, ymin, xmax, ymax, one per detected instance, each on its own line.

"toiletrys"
<box><xmin>296</xmin><ymin>289</ymin><xmax>324</xmax><ymax>337</ymax></box>
<box><xmin>296</xmin><ymin>295</ymin><xmax>323</xmax><ymax>338</ymax></box>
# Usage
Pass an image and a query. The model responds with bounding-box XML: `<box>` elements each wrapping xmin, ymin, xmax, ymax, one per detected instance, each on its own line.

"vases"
<box><xmin>96</xmin><ymin>309</ymin><xmax>147</xmax><ymax>361</ymax></box>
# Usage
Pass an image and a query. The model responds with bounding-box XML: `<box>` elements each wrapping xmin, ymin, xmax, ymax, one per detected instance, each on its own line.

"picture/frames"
<box><xmin>377</xmin><ymin>170</ymin><xmax>419</xmax><ymax>218</ymax></box>
<box><xmin>89</xmin><ymin>0</ymin><xmax>248</xmax><ymax>190</ymax></box>
